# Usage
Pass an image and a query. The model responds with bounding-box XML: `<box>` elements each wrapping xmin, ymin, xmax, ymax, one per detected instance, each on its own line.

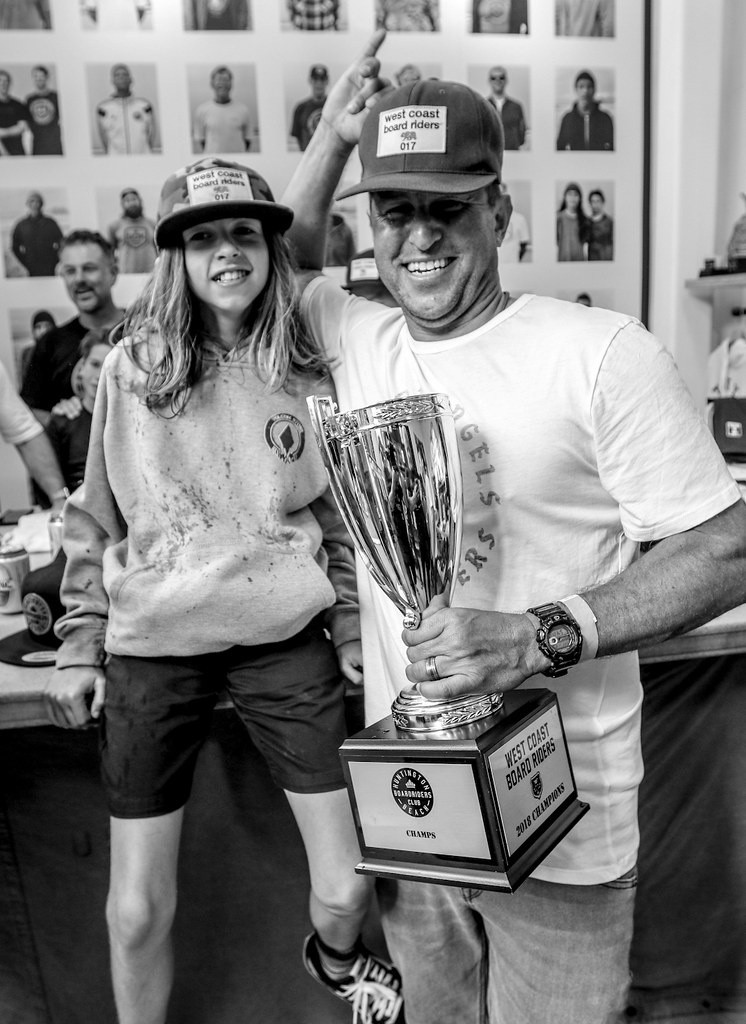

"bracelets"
<box><xmin>556</xmin><ymin>593</ymin><xmax>600</xmax><ymax>663</ymax></box>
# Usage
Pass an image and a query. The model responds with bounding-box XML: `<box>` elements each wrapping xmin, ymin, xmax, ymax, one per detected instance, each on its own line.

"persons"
<box><xmin>289</xmin><ymin>0</ymin><xmax>340</xmax><ymax>32</ymax></box>
<box><xmin>396</xmin><ymin>63</ymin><xmax>420</xmax><ymax>87</ymax></box>
<box><xmin>0</xmin><ymin>363</ymin><xmax>74</xmax><ymax>518</ymax></box>
<box><xmin>575</xmin><ymin>293</ymin><xmax>591</xmax><ymax>306</ymax></box>
<box><xmin>323</xmin><ymin>216</ymin><xmax>358</xmax><ymax>269</ymax></box>
<box><xmin>341</xmin><ymin>249</ymin><xmax>401</xmax><ymax>309</ymax></box>
<box><xmin>375</xmin><ymin>0</ymin><xmax>440</xmax><ymax>31</ymax></box>
<box><xmin>19</xmin><ymin>231</ymin><xmax>131</xmax><ymax>512</ymax></box>
<box><xmin>280</xmin><ymin>25</ymin><xmax>745</xmax><ymax>1024</ymax></box>
<box><xmin>0</xmin><ymin>0</ymin><xmax>254</xmax><ymax>360</ymax></box>
<box><xmin>32</xmin><ymin>322</ymin><xmax>119</xmax><ymax>504</ymax></box>
<box><xmin>289</xmin><ymin>62</ymin><xmax>333</xmax><ymax>150</ymax></box>
<box><xmin>43</xmin><ymin>157</ymin><xmax>406</xmax><ymax>1023</ymax></box>
<box><xmin>472</xmin><ymin>0</ymin><xmax>613</xmax><ymax>261</ymax></box>
<box><xmin>373</xmin><ymin>424</ymin><xmax>447</xmax><ymax>609</ymax></box>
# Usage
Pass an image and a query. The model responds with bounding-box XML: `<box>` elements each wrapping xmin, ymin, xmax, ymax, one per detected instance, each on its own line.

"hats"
<box><xmin>0</xmin><ymin>545</ymin><xmax>67</xmax><ymax>668</ymax></box>
<box><xmin>311</xmin><ymin>68</ymin><xmax>328</xmax><ymax>79</ymax></box>
<box><xmin>336</xmin><ymin>78</ymin><xmax>504</xmax><ymax>201</ymax></box>
<box><xmin>153</xmin><ymin>157</ymin><xmax>295</xmax><ymax>256</ymax></box>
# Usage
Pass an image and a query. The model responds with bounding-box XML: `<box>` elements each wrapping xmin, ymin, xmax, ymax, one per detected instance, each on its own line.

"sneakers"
<box><xmin>301</xmin><ymin>931</ymin><xmax>407</xmax><ymax>1024</ymax></box>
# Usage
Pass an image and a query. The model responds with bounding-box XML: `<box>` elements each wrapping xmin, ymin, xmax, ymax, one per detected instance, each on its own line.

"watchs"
<box><xmin>50</xmin><ymin>487</ymin><xmax>70</xmax><ymax>500</ymax></box>
<box><xmin>526</xmin><ymin>604</ymin><xmax>582</xmax><ymax>679</ymax></box>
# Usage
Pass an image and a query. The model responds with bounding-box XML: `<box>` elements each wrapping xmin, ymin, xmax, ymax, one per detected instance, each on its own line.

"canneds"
<box><xmin>0</xmin><ymin>544</ymin><xmax>30</xmax><ymax>615</ymax></box>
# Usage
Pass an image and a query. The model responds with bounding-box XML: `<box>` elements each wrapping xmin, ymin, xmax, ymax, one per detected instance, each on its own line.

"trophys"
<box><xmin>306</xmin><ymin>388</ymin><xmax>590</xmax><ymax>898</ymax></box>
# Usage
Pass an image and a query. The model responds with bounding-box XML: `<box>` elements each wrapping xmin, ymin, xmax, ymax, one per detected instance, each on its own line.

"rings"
<box><xmin>425</xmin><ymin>657</ymin><xmax>439</xmax><ymax>682</ymax></box>
